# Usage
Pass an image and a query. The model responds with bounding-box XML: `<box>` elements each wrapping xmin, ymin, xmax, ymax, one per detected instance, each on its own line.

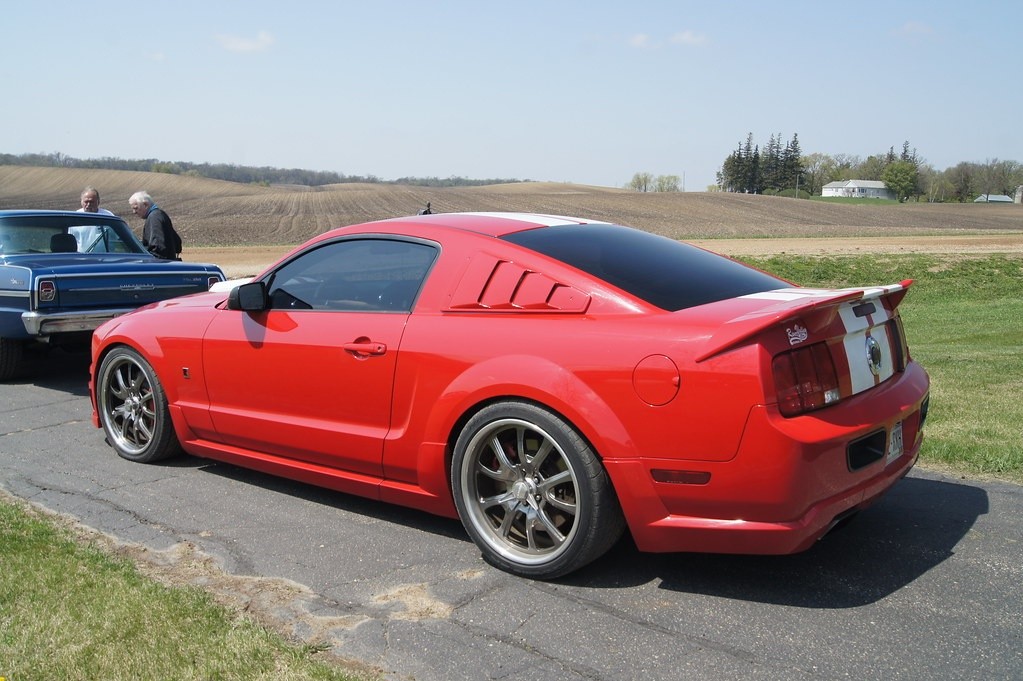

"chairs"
<box><xmin>378</xmin><ymin>277</ymin><xmax>421</xmax><ymax>311</ymax></box>
<box><xmin>50</xmin><ymin>234</ymin><xmax>77</xmax><ymax>253</ymax></box>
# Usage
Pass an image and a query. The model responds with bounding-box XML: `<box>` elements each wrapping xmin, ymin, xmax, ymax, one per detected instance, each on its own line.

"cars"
<box><xmin>1</xmin><ymin>209</ymin><xmax>228</xmax><ymax>387</ymax></box>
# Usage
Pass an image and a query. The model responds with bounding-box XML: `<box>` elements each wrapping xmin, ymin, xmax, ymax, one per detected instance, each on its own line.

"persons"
<box><xmin>67</xmin><ymin>186</ymin><xmax>122</xmax><ymax>254</ymax></box>
<box><xmin>128</xmin><ymin>191</ymin><xmax>183</xmax><ymax>261</ymax></box>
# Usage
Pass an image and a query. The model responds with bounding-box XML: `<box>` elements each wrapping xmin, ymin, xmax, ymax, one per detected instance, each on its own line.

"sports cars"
<box><xmin>87</xmin><ymin>208</ymin><xmax>930</xmax><ymax>583</ymax></box>
